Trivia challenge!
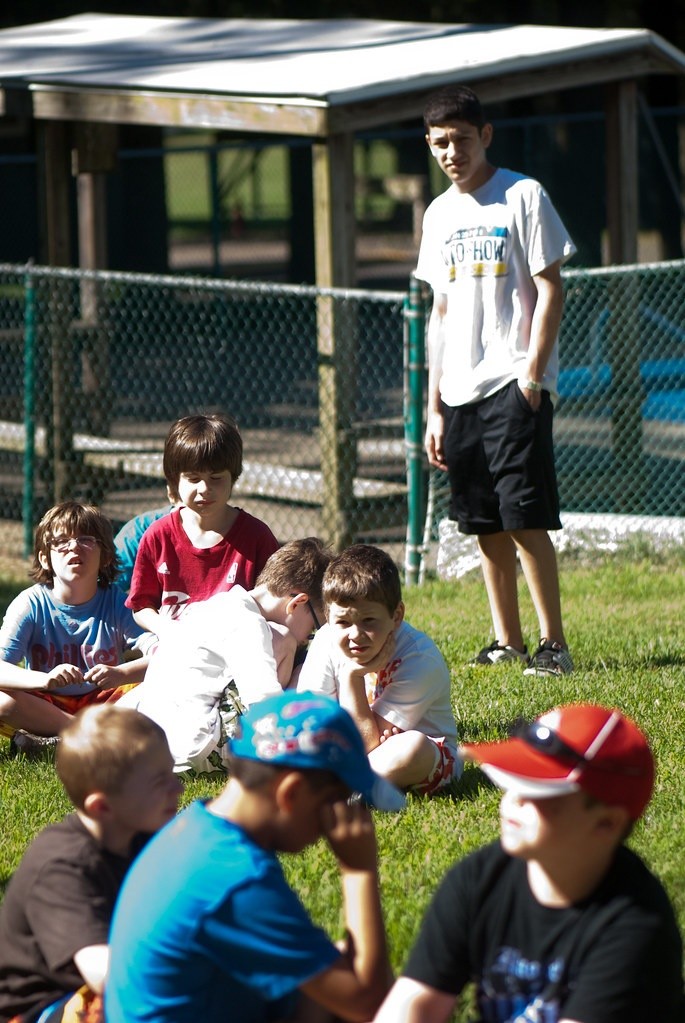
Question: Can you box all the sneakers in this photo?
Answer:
[523,637,573,678]
[462,640,530,669]
[13,728,62,764]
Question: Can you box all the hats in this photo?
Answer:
[461,703,655,822]
[229,689,407,813]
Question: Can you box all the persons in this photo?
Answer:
[102,691,395,1023]
[0,702,185,1023]
[0,502,158,765]
[373,703,685,1023]
[415,88,578,675]
[296,545,463,797]
[114,482,183,593]
[137,538,336,782]
[123,414,282,639]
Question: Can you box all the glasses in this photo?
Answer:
[47,536,107,552]
[506,717,643,777]
[290,593,321,640]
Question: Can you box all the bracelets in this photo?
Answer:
[517,379,541,392]
